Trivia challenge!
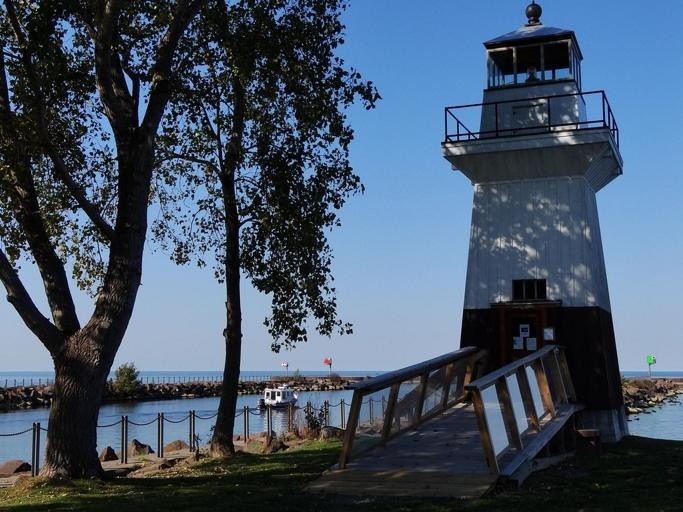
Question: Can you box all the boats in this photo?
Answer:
[258,384,298,410]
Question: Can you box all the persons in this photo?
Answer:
[525,66,540,83]
